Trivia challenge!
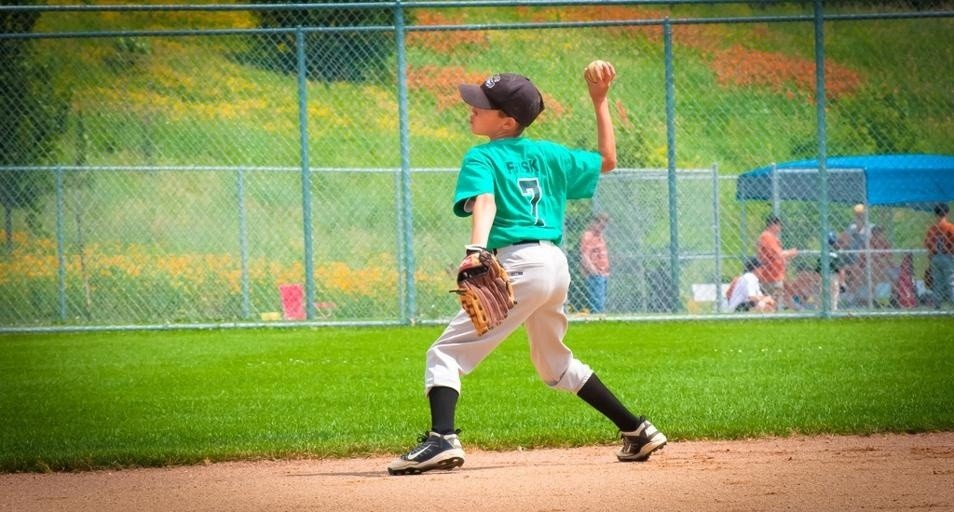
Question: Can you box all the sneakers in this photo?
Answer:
[616,415,667,462]
[388,429,465,475]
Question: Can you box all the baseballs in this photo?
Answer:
[588,61,610,83]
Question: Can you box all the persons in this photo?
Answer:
[925,204,954,311]
[580,213,610,314]
[728,212,900,313]
[388,62,667,476]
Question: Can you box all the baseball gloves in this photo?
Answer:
[457,244,515,335]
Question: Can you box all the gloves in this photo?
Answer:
[840,282,848,293]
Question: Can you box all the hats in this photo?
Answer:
[596,213,608,226]
[744,256,763,271]
[459,73,545,127]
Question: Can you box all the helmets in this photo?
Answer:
[854,204,866,214]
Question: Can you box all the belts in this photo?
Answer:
[513,240,539,245]
